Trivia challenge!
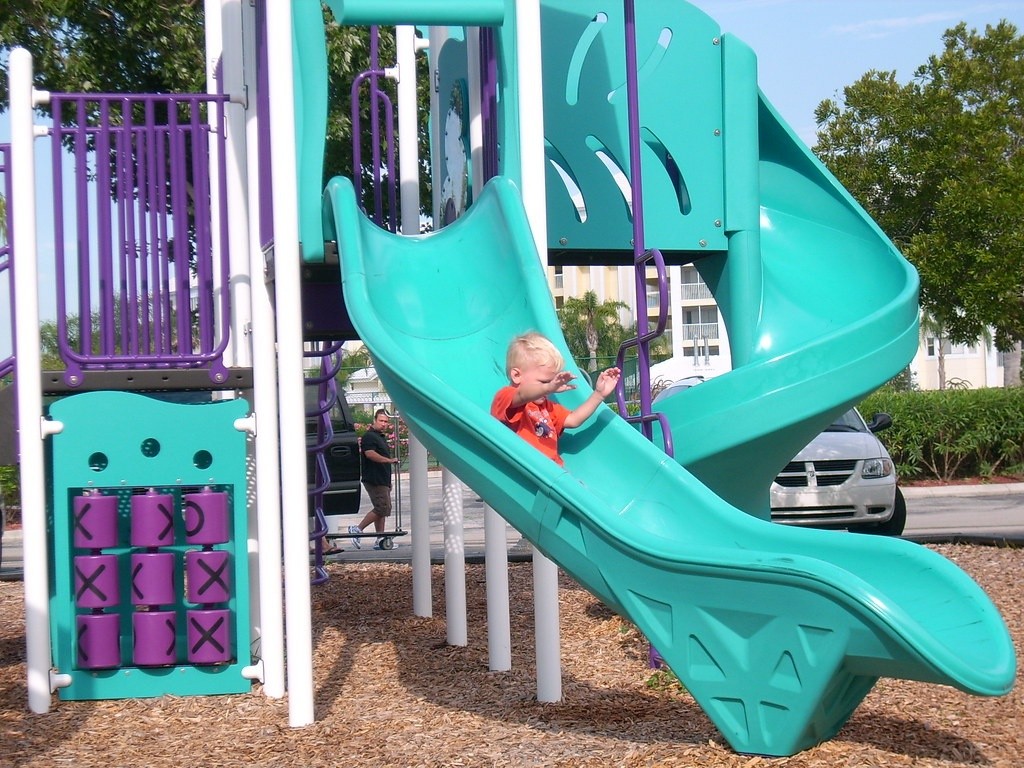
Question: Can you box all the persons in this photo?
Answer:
[347,409,400,550]
[309,516,344,555]
[490,333,621,468]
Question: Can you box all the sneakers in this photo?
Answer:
[373,538,400,549]
[348,524,364,549]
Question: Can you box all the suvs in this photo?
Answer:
[645,374,907,539]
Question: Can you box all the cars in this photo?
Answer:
[143,381,361,516]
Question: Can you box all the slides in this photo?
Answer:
[329,170,1020,759]
[630,82,927,523]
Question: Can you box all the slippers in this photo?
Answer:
[323,547,345,555]
